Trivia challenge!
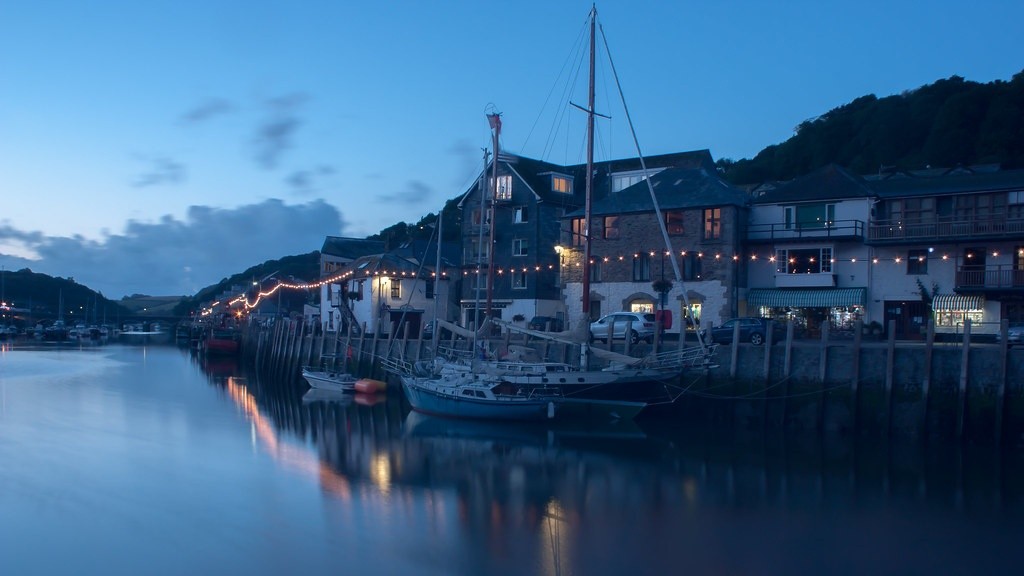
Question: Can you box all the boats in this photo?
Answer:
[303,372,360,393]
[377,333,726,421]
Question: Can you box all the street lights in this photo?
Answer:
[552,224,590,255]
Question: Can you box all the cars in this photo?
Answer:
[996,322,1024,341]
[424,320,465,339]
[589,311,663,344]
[697,316,785,346]
